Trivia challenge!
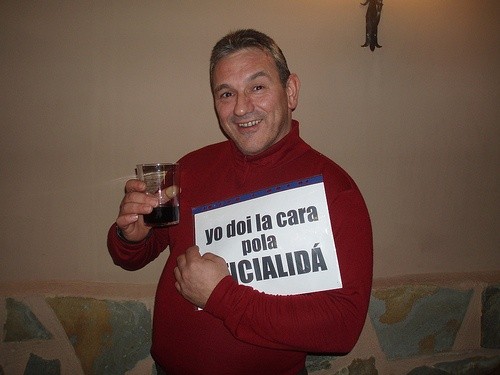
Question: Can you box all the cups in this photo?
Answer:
[135,163,180,227]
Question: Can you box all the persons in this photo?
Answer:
[107,27,373,375]
